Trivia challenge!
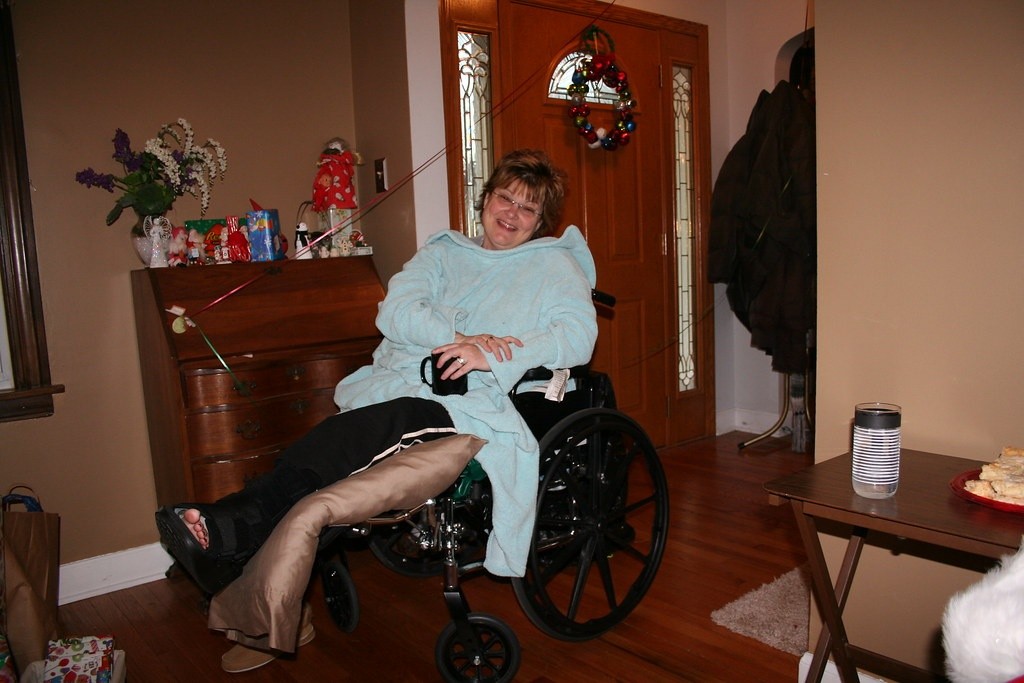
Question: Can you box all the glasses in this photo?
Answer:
[492,191,542,217]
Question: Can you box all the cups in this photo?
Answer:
[847,404,903,500]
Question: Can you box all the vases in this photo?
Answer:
[130,213,175,269]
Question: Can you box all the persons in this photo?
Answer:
[152,151,601,601]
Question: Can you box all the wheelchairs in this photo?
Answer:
[314,290,670,683]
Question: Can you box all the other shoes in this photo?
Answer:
[155,461,316,595]
[221,604,316,673]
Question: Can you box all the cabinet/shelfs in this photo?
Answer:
[129,253,388,510]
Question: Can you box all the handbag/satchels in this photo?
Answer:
[2,484,61,641]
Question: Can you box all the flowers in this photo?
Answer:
[75,118,227,226]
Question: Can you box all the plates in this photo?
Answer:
[948,464,1024,512]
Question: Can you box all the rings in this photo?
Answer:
[486,337,493,343]
[456,358,465,365]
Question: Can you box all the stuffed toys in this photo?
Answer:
[311,139,357,212]
[168,213,248,266]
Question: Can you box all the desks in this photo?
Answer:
[760,447,1024,683]
